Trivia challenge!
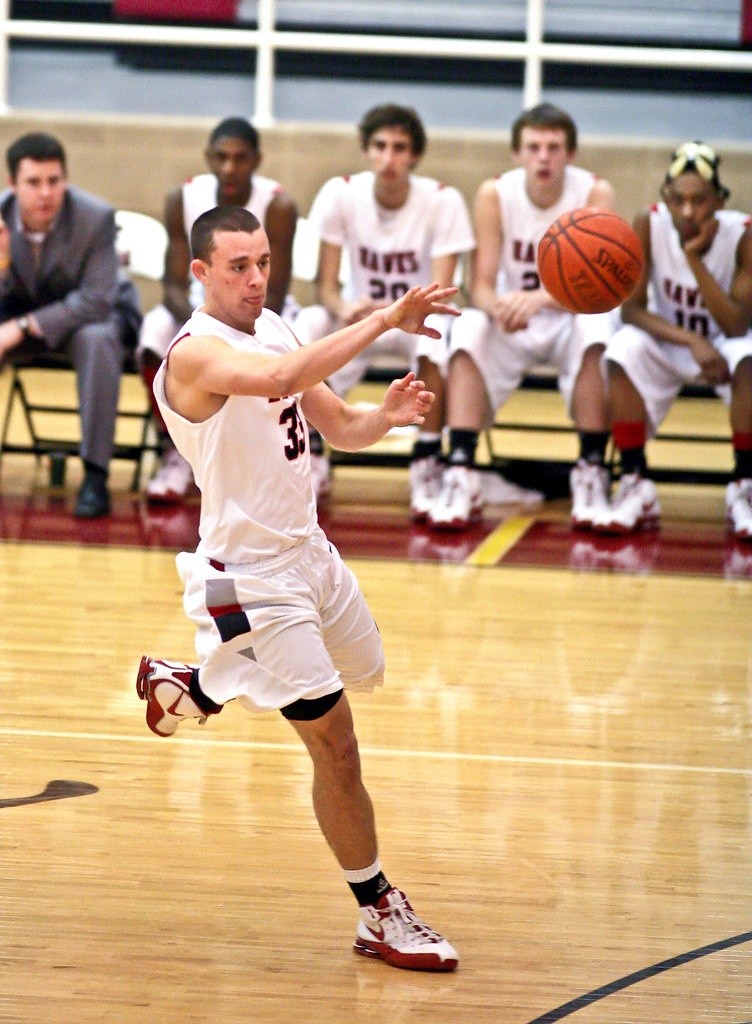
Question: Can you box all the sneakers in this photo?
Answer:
[311,454,331,499]
[570,462,611,525]
[411,459,444,514]
[591,475,660,531]
[145,450,194,500]
[724,478,752,537]
[135,655,224,738]
[429,465,481,527]
[353,887,459,970]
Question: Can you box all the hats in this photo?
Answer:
[665,142,720,188]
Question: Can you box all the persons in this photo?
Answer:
[297,104,616,532]
[136,205,462,973]
[0,116,297,520]
[608,141,752,542]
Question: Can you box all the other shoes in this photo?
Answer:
[71,475,108,516]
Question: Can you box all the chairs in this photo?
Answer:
[0,209,738,501]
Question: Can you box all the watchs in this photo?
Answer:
[16,314,30,338]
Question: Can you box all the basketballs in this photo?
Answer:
[537,205,645,315]
